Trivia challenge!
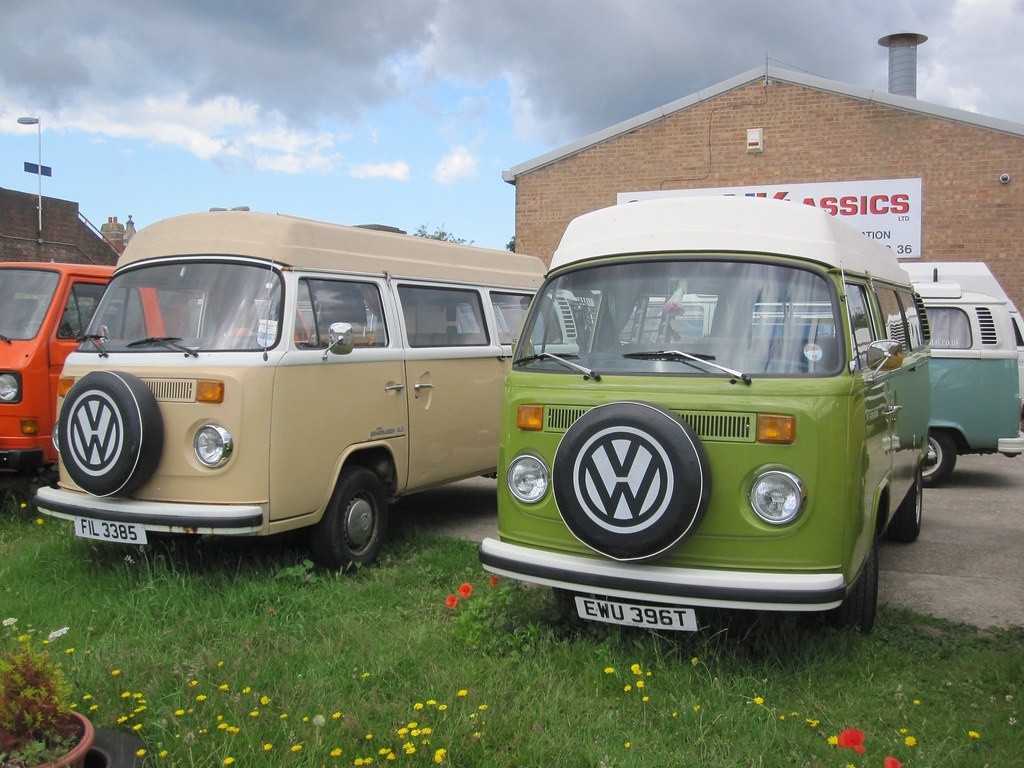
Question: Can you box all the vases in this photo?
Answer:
[0,709,95,768]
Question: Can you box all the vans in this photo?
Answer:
[35,211,560,573]
[1,260,309,482]
[471,195,935,635]
[616,281,1021,489]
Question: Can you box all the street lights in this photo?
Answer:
[16,117,44,244]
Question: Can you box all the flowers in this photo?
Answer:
[0,617,70,752]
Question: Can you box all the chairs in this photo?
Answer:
[400,303,416,347]
[416,304,449,348]
